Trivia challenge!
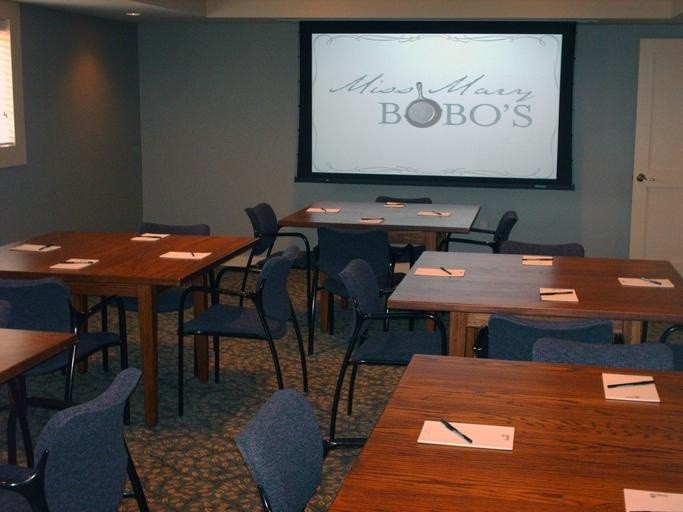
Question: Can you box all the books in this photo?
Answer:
[623,487,683,512]
[305,201,451,224]
[601,372,661,402]
[411,253,676,303]
[8,232,213,271]
[417,420,515,450]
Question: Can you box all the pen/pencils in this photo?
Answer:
[39,244,53,250]
[385,204,405,205]
[361,218,385,220]
[540,292,573,295]
[190,249,194,256]
[641,277,661,285]
[440,419,472,443]
[440,267,451,275]
[522,259,553,260]
[136,234,162,238]
[321,208,327,212]
[61,261,92,264]
[432,210,442,216]
[607,381,655,388]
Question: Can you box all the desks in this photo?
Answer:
[326,353,683,512]
[276,199,481,335]
[386,248,683,358]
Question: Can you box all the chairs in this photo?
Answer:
[533,335,677,370]
[438,209,519,315]
[486,312,614,361]
[501,239,585,257]
